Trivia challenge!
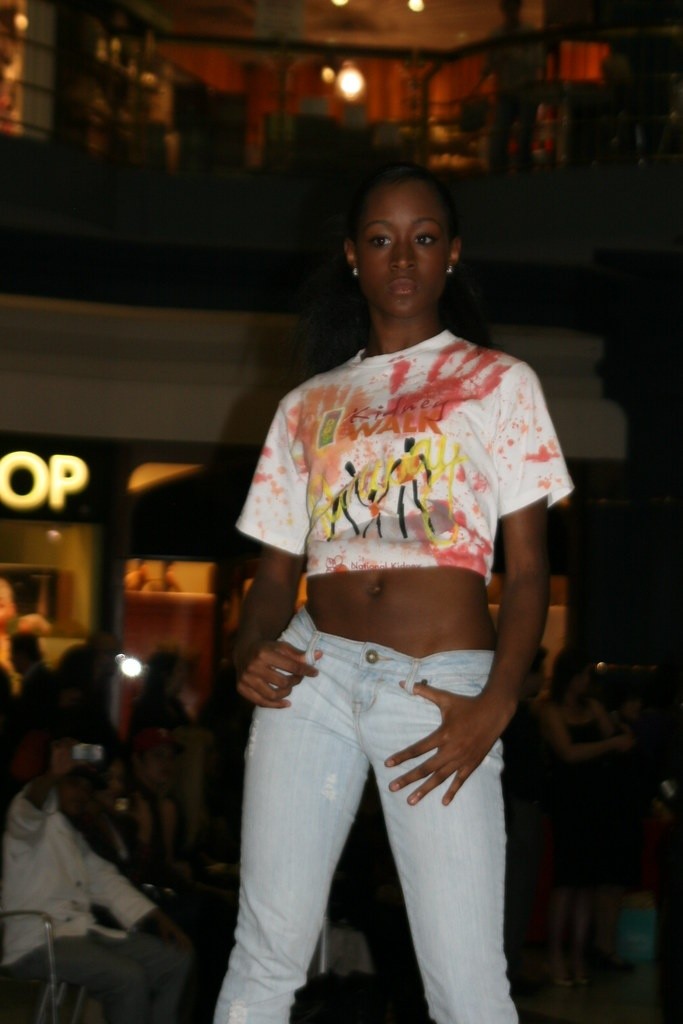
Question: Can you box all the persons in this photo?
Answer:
[211,172,575,1024]
[0,633,402,1024]
[486,0,544,172]
[506,647,683,984]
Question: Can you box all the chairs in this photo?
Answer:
[0,909,88,1024]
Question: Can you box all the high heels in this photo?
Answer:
[542,957,572,987]
[574,973,588,985]
